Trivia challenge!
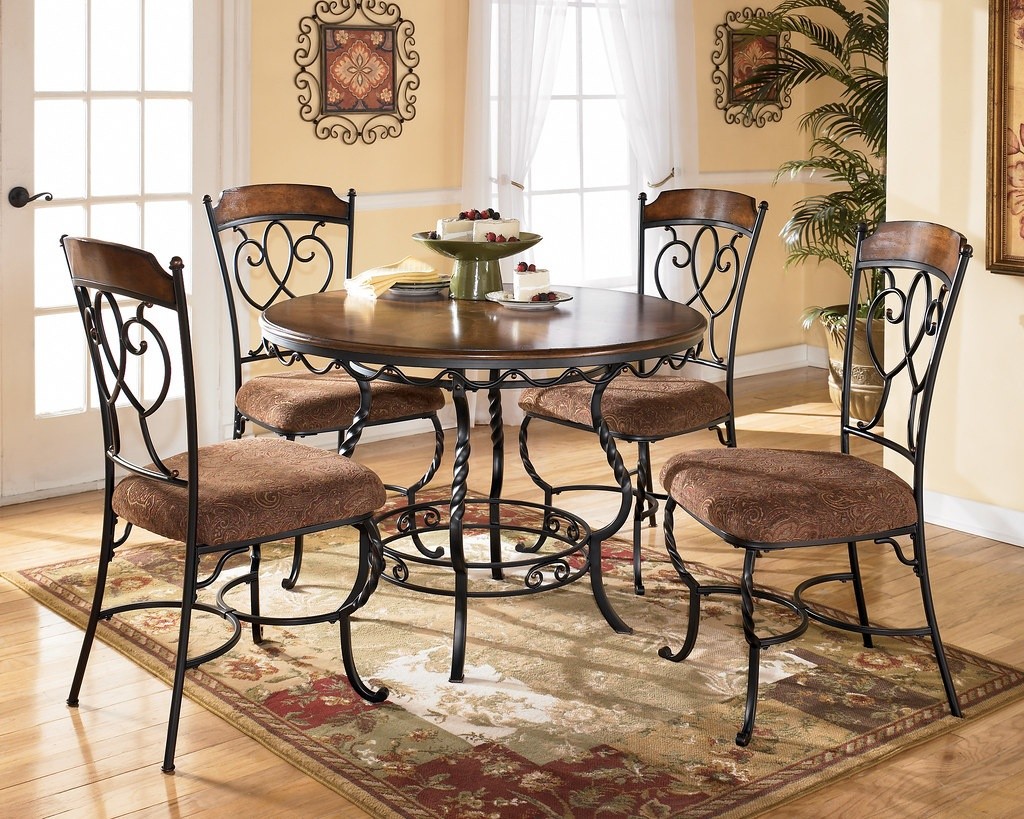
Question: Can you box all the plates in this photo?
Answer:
[388,273,450,296]
[485,290,573,310]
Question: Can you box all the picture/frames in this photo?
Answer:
[986,0,1024,277]
[710,6,794,129]
[294,1,420,146]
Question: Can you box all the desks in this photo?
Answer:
[257,277,707,682]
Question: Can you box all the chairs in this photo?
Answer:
[57,234,388,774]
[514,190,771,638]
[203,182,446,592]
[658,216,973,751]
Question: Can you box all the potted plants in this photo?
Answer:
[728,0,886,424]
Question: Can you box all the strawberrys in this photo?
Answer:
[485,232,520,242]
[532,292,556,301]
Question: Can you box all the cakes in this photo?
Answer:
[436,208,520,242]
[513,262,550,301]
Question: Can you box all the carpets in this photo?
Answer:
[1,480,1024,819]
